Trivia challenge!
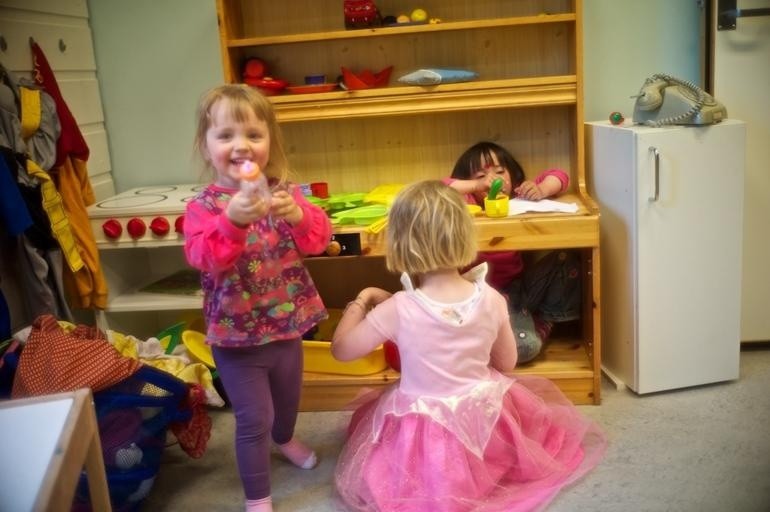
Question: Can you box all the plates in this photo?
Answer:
[290,84,338,95]
[181,329,216,369]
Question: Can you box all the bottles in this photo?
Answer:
[239,159,272,212]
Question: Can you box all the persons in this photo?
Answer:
[438,141,583,347]
[179,84,336,512]
[329,181,609,512]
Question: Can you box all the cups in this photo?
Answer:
[344,0,377,28]
[484,194,511,217]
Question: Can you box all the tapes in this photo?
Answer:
[305,75,325,85]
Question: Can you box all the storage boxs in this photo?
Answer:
[302,307,387,375]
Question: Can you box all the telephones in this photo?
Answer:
[633,74,728,125]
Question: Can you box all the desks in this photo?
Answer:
[0,387,113,512]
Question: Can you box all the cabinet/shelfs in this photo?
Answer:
[86,184,205,333]
[214,1,601,417]
[584,116,745,395]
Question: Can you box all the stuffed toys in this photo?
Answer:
[501,307,542,365]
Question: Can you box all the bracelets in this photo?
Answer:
[355,296,370,306]
